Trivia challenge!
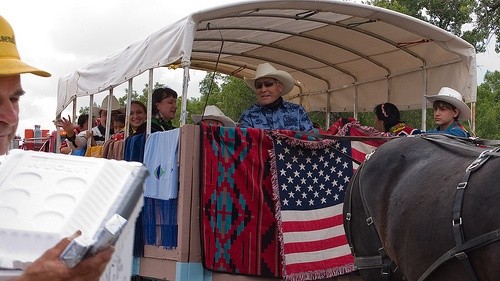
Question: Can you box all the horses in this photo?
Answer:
[342,130,500,281]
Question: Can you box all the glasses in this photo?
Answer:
[254,80,276,89]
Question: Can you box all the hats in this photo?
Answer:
[424,87,471,120]
[0,14,52,78]
[243,63,294,95]
[92,94,125,117]
[191,105,236,127]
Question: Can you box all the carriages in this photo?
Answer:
[54,0,500,280]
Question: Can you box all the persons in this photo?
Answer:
[111,101,147,140]
[113,114,125,134]
[55,94,121,150]
[193,105,236,127]
[423,87,472,138]
[133,88,177,136]
[0,16,114,281]
[374,103,421,136]
[235,63,314,132]
